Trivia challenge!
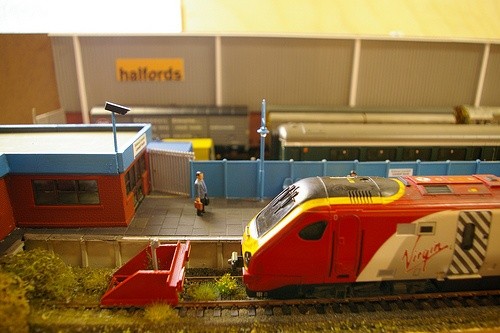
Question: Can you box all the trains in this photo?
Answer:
[242,174,500,296]
[279,123,500,162]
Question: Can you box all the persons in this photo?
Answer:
[194,171,207,217]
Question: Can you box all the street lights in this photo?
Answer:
[259,99,267,202]
